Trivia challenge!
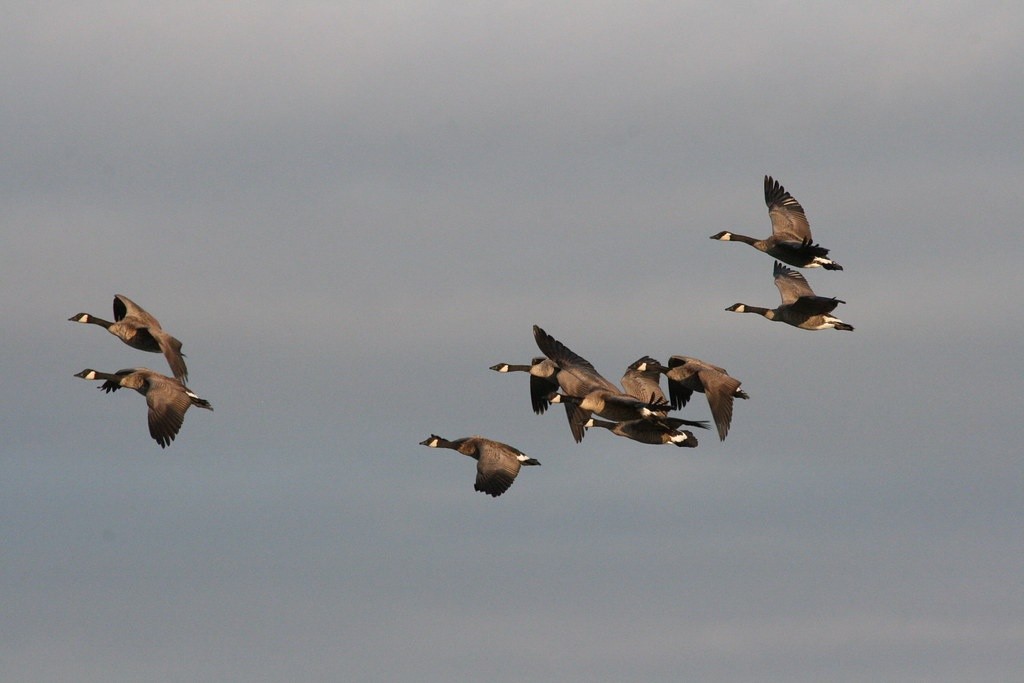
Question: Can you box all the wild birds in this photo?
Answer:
[418,431,544,497]
[68,291,213,449]
[709,172,855,332]
[488,322,750,448]
